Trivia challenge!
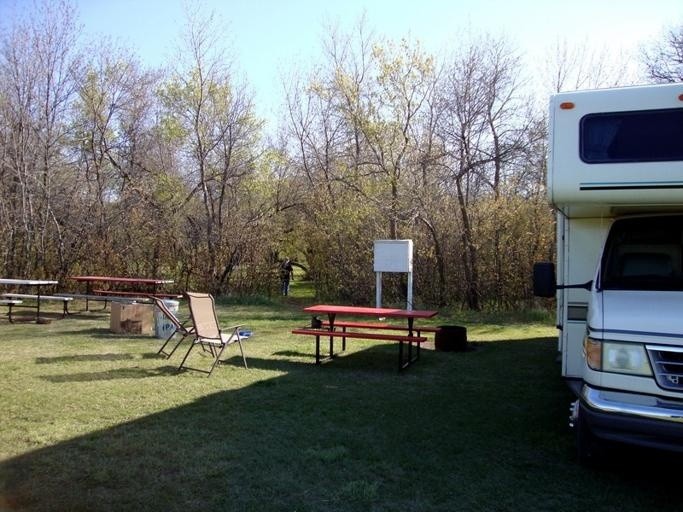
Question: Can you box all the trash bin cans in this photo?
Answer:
[153,300,179,339]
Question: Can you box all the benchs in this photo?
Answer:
[0,292,73,319]
[290,328,426,374]
[52,293,150,316]
[0,299,24,325]
[92,288,184,310]
[321,320,442,360]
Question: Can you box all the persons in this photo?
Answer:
[277,257,294,296]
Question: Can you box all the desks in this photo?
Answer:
[67,275,175,312]
[304,304,438,373]
[0,278,58,322]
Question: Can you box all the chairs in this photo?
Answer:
[176,290,248,378]
[148,294,214,360]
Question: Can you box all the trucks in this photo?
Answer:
[534,82,682,453]
